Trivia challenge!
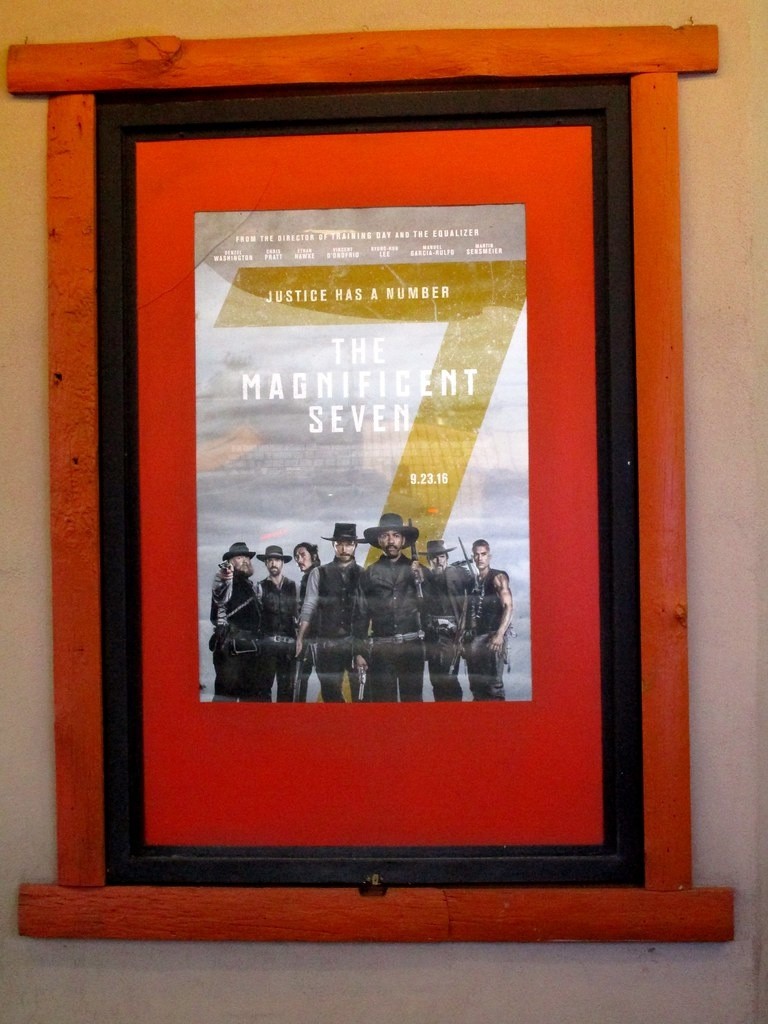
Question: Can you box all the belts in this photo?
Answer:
[372,631,420,644]
[262,635,296,644]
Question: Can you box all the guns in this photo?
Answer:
[357,666,368,701]
[218,560,230,572]
[288,517,510,702]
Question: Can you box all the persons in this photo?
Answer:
[352,514,449,702]
[251,545,298,702]
[460,539,514,701]
[291,542,322,699]
[209,543,263,701]
[419,540,474,701]
[295,522,372,703]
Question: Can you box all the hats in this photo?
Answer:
[418,539,457,556]
[320,523,368,543]
[364,513,420,549]
[256,545,293,563]
[222,542,256,562]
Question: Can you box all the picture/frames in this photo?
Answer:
[3,25,734,946]
